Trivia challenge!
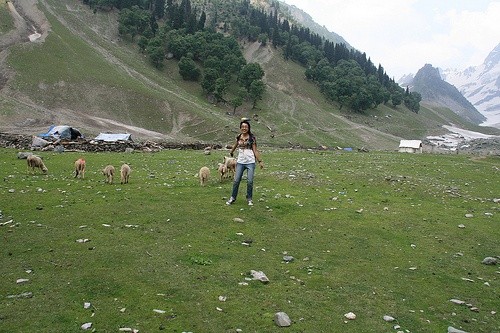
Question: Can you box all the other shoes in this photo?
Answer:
[226,197,235,204]
[248,200,253,206]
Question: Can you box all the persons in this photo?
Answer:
[226,121,264,206]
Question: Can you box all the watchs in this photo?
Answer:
[258,161,261,163]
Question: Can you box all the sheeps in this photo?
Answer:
[102,164,116,185]
[119,163,131,184]
[27,154,48,174]
[216,156,237,183]
[198,166,211,186]
[73,157,86,180]
